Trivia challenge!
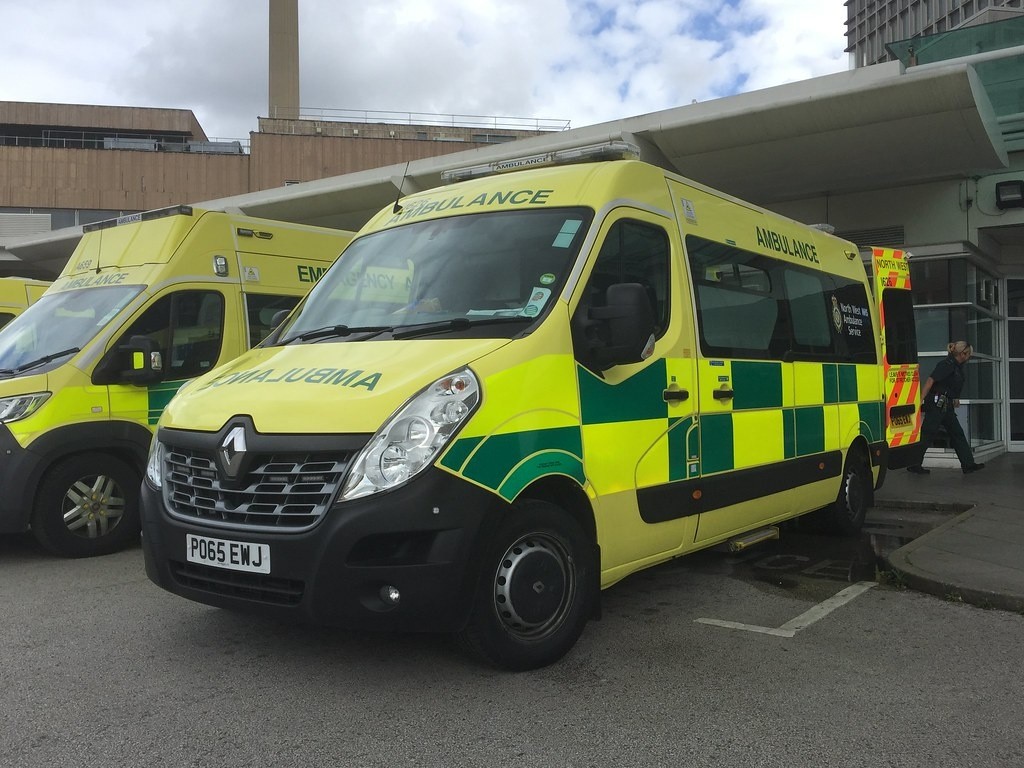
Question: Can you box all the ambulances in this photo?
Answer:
[1,276,97,371]
[1,202,415,560]
[137,141,923,672]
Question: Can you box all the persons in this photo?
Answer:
[906,340,985,475]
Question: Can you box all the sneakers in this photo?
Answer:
[907,465,930,474]
[962,463,984,474]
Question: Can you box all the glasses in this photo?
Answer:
[962,343,970,352]
